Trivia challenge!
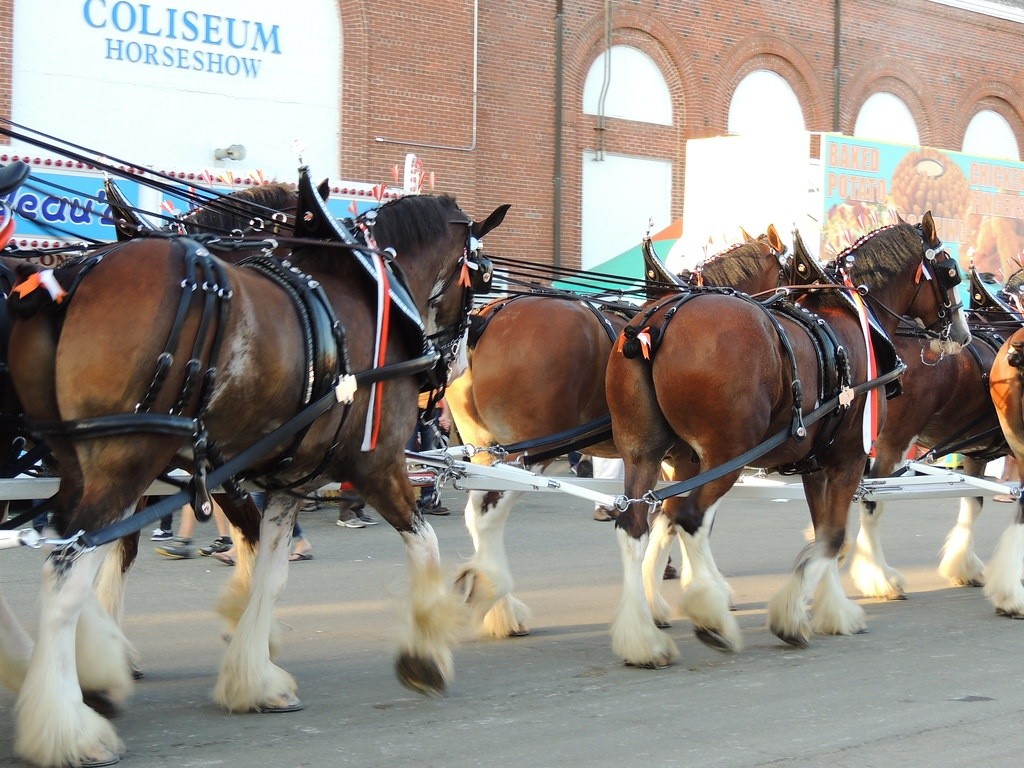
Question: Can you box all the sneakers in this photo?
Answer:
[422,504,450,514]
[150,527,174,540]
[156,540,196,559]
[358,515,379,525]
[196,537,233,557]
[336,518,367,528]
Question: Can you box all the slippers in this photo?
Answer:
[288,552,314,561]
[213,553,236,566]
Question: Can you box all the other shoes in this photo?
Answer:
[19,528,44,547]
[571,463,579,475]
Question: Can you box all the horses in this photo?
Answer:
[0,178,1024,768]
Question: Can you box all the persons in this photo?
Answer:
[568,450,621,521]
[32,461,58,533]
[150,491,379,567]
[418,387,451,520]
[0,159,30,198]
